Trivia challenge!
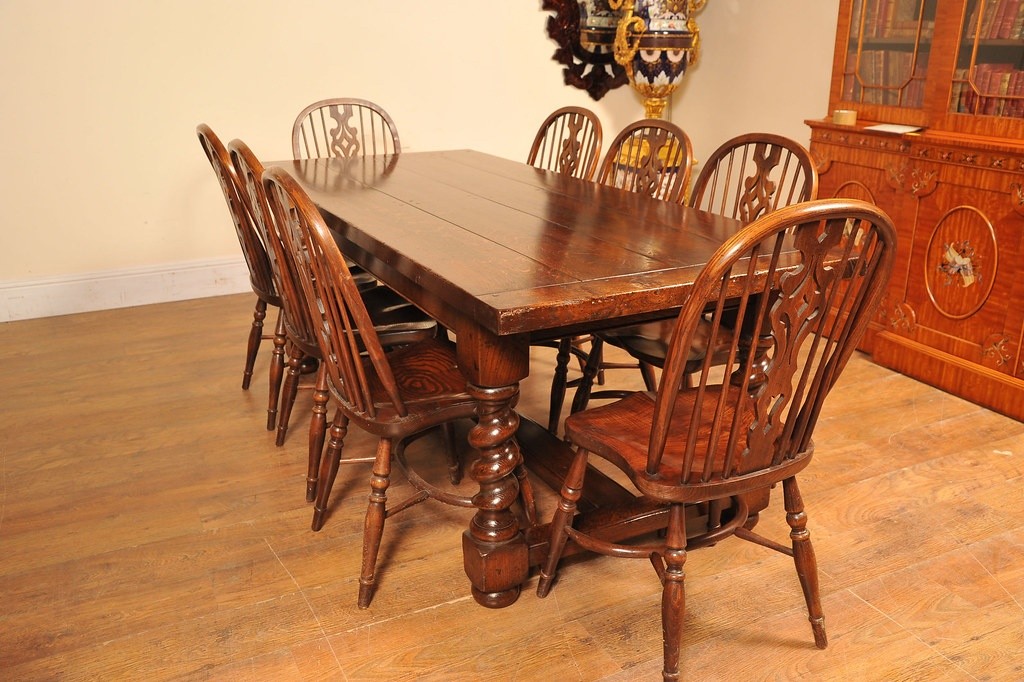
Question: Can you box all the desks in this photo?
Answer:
[269,145,864,610]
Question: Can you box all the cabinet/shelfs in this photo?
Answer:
[782,0,1024,425]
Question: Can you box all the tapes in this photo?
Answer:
[832,109,858,126]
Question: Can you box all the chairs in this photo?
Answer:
[194,98,897,681]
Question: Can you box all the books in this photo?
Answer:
[948,0,1024,117]
[841,0,935,108]
